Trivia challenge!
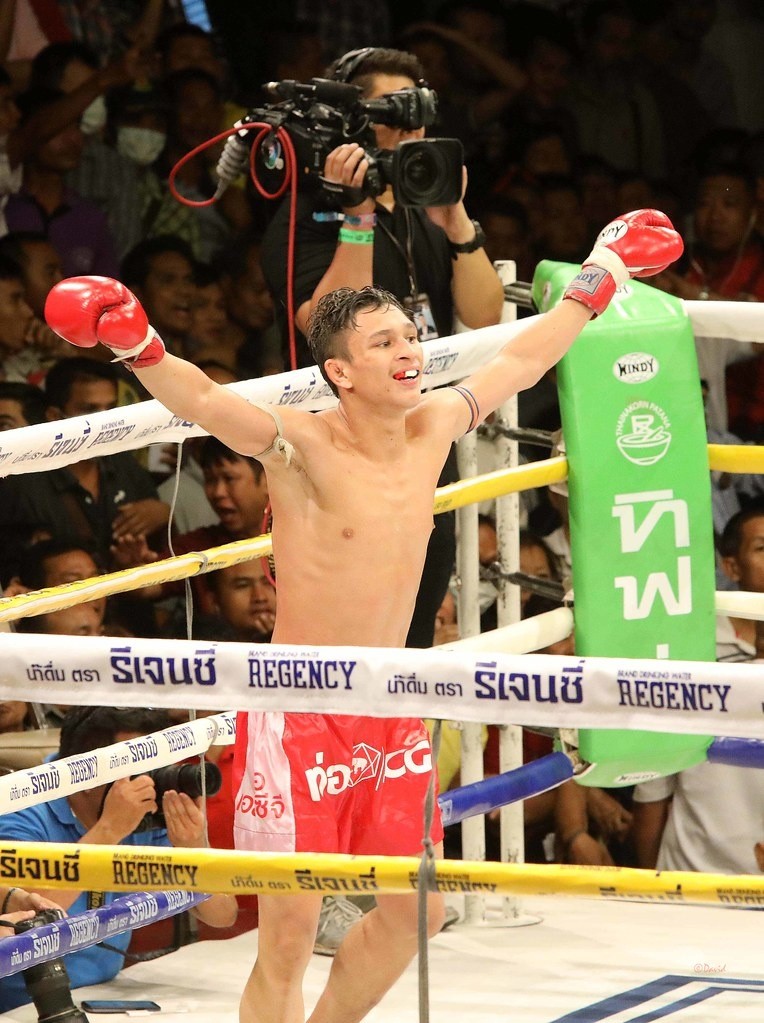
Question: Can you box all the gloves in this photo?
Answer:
[564,207,684,320]
[45,274,166,370]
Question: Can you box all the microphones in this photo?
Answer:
[266,76,364,106]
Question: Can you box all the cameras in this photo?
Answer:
[12,907,90,1023]
[126,758,222,833]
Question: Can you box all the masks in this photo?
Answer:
[118,127,167,163]
[79,96,108,133]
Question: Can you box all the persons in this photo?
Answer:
[257,48,506,960]
[0,0,764,964]
[0,706,238,1014]
[45,207,684,1021]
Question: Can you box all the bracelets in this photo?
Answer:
[342,214,377,226]
[3,888,15,914]
[696,285,710,300]
[337,228,374,244]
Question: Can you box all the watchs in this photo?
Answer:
[447,220,487,261]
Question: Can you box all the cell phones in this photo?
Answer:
[81,999,162,1013]
[177,0,214,34]
